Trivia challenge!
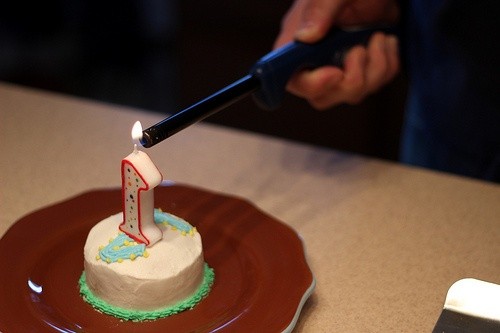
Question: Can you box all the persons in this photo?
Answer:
[274,1,499,184]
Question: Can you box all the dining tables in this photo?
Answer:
[0,85,500,332]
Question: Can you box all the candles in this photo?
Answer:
[118,119,163,247]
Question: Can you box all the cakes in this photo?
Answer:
[79,205,215,323]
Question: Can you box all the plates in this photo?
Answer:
[0,180,314,333]
